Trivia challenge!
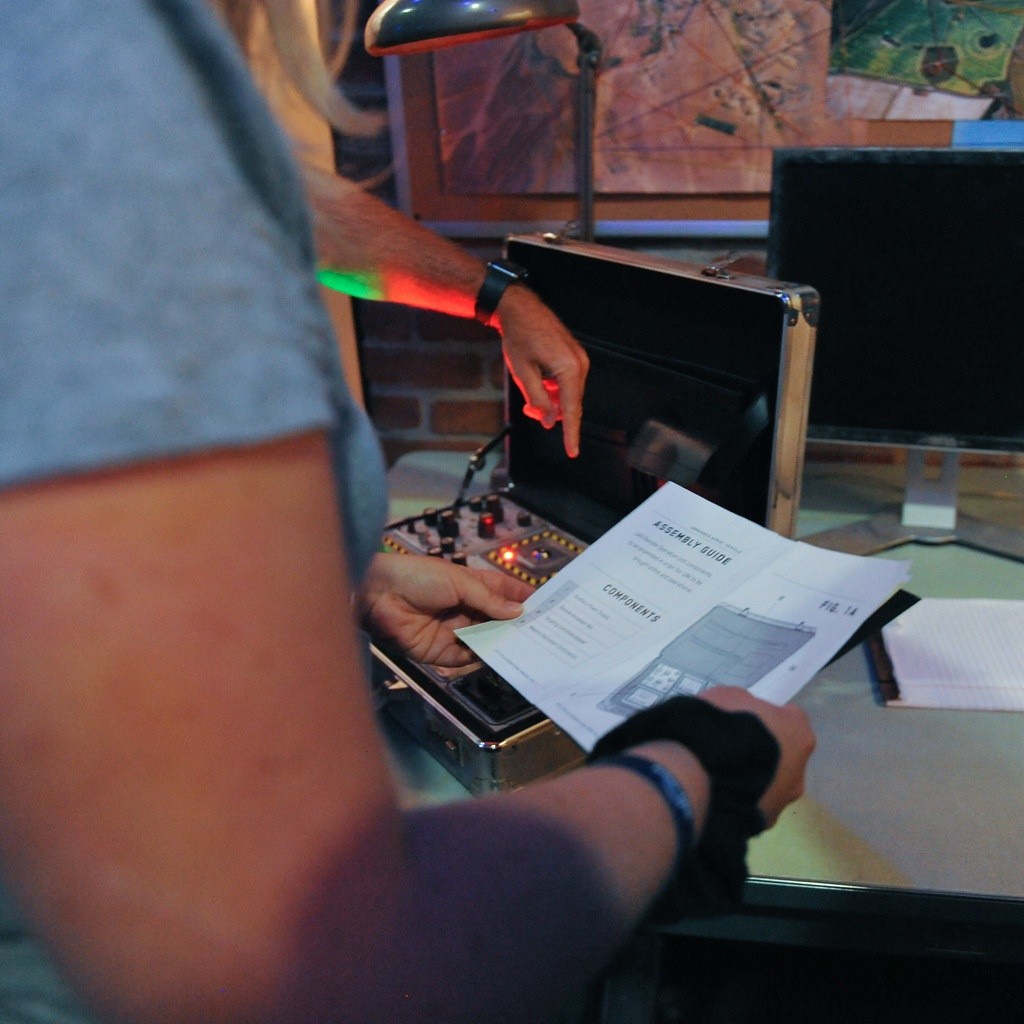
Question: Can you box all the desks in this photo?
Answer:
[382,450,1024,927]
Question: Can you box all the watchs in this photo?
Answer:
[475,256,529,325]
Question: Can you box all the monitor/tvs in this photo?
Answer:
[767,140,1024,568]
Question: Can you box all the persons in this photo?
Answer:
[0,0,815,1024]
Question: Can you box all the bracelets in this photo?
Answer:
[591,691,783,890]
[581,745,702,880]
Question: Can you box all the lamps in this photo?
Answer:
[363,0,603,241]
